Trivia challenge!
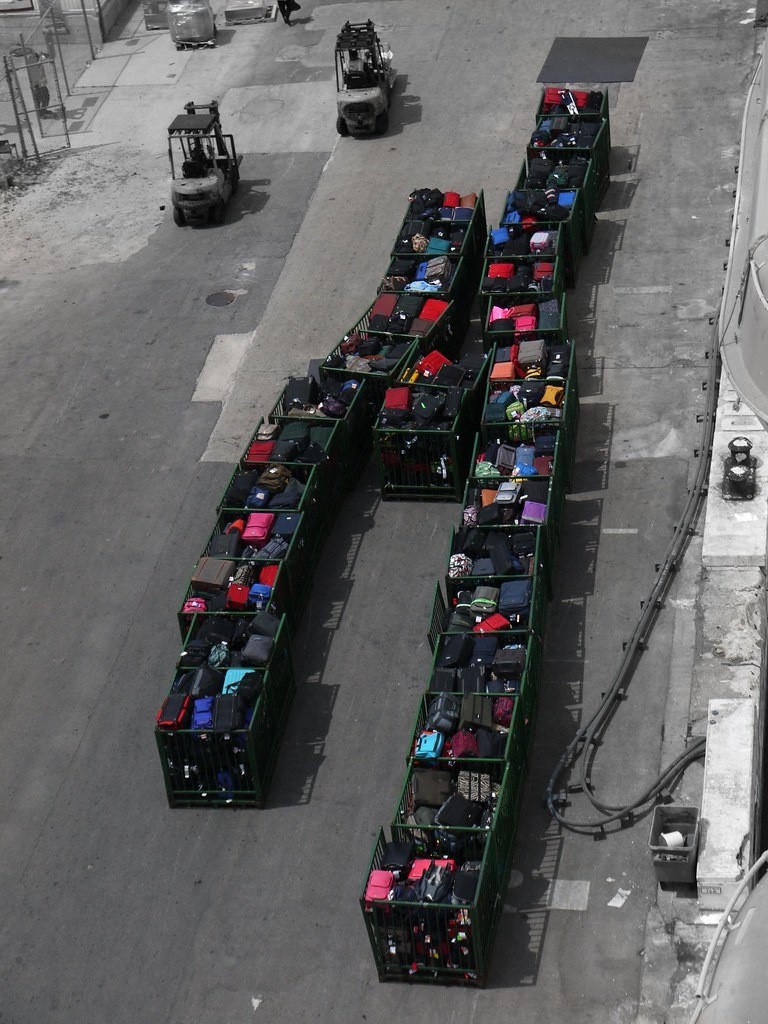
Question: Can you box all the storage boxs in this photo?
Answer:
[152,84,614,991]
[648,804,700,890]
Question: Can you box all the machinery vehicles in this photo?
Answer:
[334,18,398,136]
[166,100,243,228]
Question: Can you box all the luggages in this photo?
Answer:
[152,84,612,992]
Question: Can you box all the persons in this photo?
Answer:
[26,52,53,120]
[275,0,302,26]
[345,50,369,78]
